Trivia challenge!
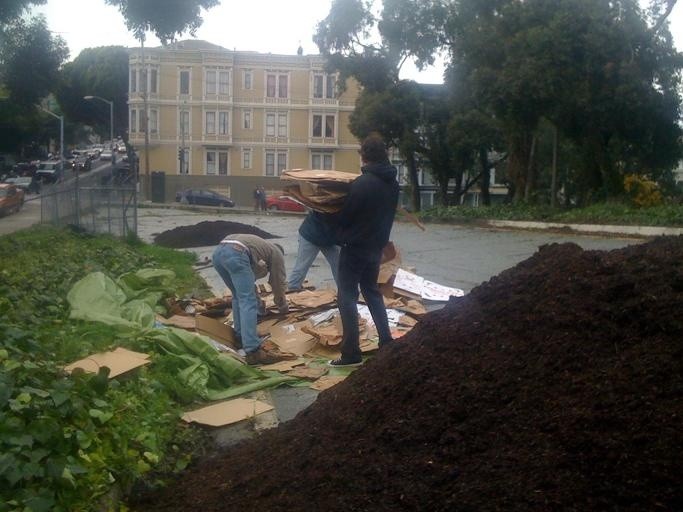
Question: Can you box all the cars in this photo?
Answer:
[0,182,25,216]
[175,185,236,207]
[4,134,127,192]
[265,193,306,213]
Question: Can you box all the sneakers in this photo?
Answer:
[245,349,282,366]
[325,355,362,367]
[234,336,242,349]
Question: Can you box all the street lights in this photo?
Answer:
[84,95,114,163]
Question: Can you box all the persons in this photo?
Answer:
[288,210,341,292]
[254,185,267,211]
[327,134,400,367]
[212,232,288,365]
